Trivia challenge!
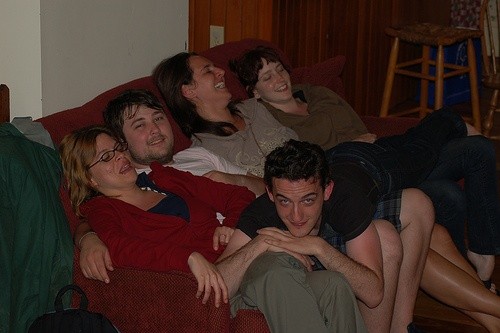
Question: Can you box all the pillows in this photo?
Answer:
[292,55,346,86]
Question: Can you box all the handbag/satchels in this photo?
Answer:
[28,284,120,333]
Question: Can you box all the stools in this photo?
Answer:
[379,23,483,133]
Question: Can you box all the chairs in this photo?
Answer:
[480,0,500,141]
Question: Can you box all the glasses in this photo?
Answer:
[86,140,128,171]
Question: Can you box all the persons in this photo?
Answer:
[58,45,500,333]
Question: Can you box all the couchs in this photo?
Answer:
[0,38,476,333]
